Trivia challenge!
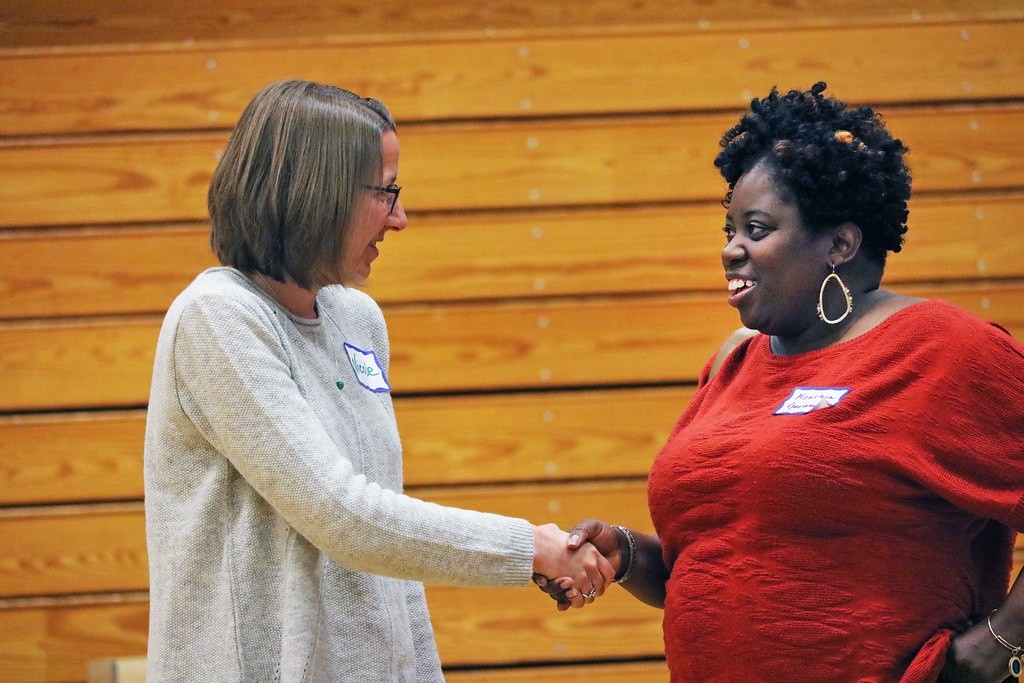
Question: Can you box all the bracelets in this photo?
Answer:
[608,525,638,585]
[987,609,1024,678]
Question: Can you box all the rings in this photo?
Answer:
[582,587,596,599]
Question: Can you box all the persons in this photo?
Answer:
[534,88,1024,683]
[143,79,615,683]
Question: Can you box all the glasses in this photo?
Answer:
[363,184,402,214]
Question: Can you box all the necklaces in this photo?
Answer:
[257,271,290,310]
